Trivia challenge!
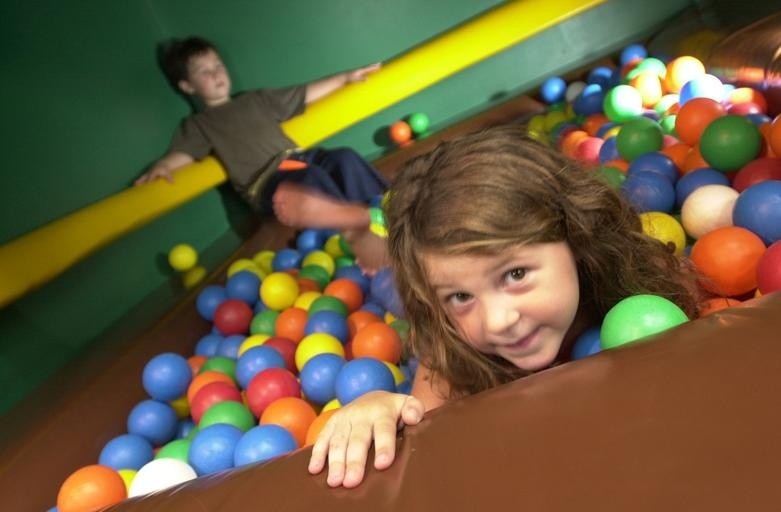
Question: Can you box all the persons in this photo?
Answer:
[132,35,382,232]
[308,126,714,489]
[525,43,781,361]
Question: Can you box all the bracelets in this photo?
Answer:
[346,71,352,85]
